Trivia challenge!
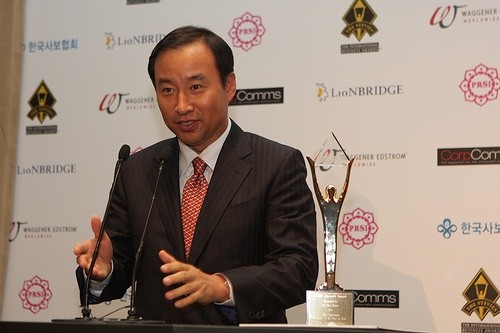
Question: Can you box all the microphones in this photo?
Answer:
[83,144,130,319]
[128,149,171,321]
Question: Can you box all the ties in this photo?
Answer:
[181,158,210,262]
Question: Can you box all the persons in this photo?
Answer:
[74,25,319,327]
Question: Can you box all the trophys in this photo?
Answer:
[306,130,356,326]
